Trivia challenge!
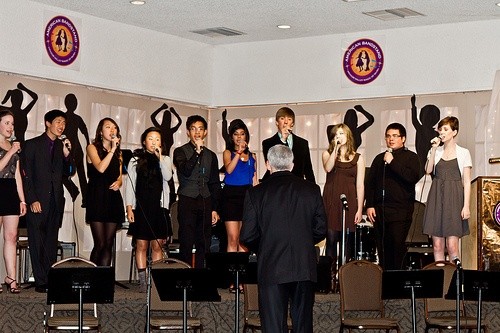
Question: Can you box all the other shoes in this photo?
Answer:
[138,271,147,293]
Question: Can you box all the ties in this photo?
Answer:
[49,142,54,160]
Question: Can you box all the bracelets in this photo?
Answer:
[110,150,115,153]
[237,151,242,154]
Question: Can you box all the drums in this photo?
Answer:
[349,214,377,261]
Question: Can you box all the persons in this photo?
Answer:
[86,117,126,267]
[365,122,425,270]
[262,106,316,182]
[0,105,27,293]
[172,114,220,267]
[220,124,258,294]
[238,144,327,333]
[124,127,173,291]
[322,123,365,293]
[20,109,75,293]
[424,115,472,265]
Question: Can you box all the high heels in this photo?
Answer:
[5,276,21,294]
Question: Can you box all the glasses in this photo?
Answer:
[386,134,403,139]
[188,127,205,131]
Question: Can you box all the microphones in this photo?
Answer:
[337,138,340,144]
[10,136,21,157]
[452,255,463,271]
[340,194,349,212]
[196,137,204,150]
[384,148,393,165]
[431,135,443,145]
[288,129,293,135]
[112,135,119,146]
[154,147,159,152]
[239,139,249,150]
[61,135,70,151]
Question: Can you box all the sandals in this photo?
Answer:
[229,287,245,294]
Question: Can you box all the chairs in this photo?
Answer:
[43,257,101,333]
[405,202,436,269]
[129,236,152,285]
[144,258,204,333]
[243,285,293,333]
[167,201,197,250]
[338,260,402,333]
[16,215,64,288]
[422,261,485,333]
